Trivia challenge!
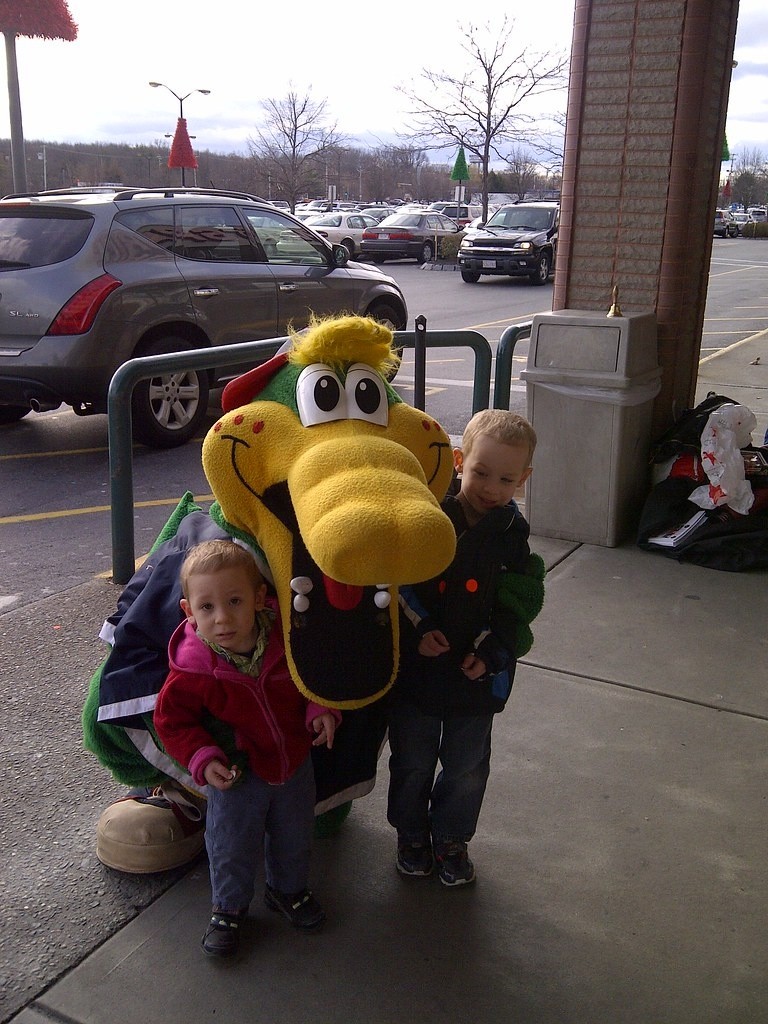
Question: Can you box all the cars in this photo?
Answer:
[217,212,294,255]
[731,206,767,232]
[359,210,457,263]
[276,213,379,261]
[293,199,516,223]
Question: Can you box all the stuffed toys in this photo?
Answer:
[82,320,453,876]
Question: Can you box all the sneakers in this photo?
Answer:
[432,832,475,887]
[265,881,327,933]
[396,828,433,876]
[200,905,248,958]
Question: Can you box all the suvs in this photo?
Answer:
[714,210,740,239]
[0,189,406,449]
[265,199,291,213]
[441,206,493,228]
[457,198,561,284]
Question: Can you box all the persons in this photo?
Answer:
[154,539,343,956]
[383,408,545,886]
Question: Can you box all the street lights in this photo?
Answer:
[164,134,197,185]
[325,137,361,198]
[149,81,210,186]
[449,125,477,225]
